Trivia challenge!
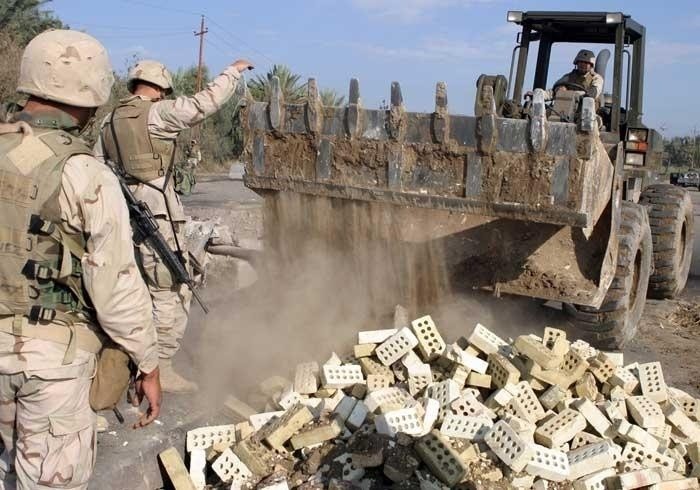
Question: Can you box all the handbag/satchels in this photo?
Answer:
[89,347,130,412]
[174,166,196,196]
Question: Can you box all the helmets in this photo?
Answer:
[127,59,174,95]
[15,29,115,107]
[573,49,595,68]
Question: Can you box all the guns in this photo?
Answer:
[108,160,208,316]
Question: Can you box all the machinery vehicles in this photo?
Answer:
[239,12,695,349]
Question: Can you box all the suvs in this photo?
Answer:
[670,168,700,192]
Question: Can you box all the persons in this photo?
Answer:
[92,59,255,395]
[185,140,201,174]
[554,50,603,113]
[0,28,161,490]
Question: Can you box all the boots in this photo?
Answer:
[157,358,198,395]
